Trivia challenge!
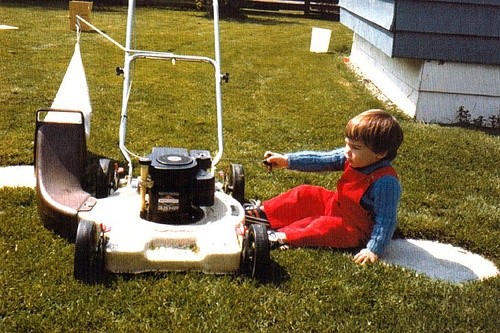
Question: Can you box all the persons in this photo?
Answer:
[243,108,405,267]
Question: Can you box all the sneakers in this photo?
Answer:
[243,198,267,226]
[250,229,280,250]
